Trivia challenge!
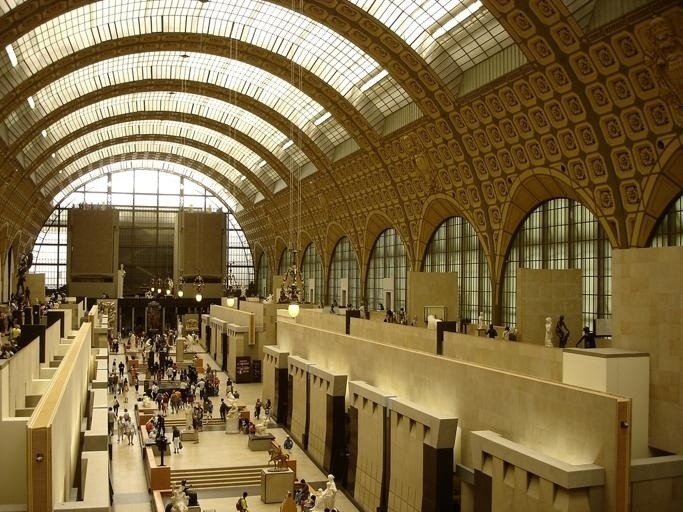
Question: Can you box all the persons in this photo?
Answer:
[501,326,510,340]
[107,323,272,454]
[294,478,316,511]
[331,509,336,512]
[574,326,597,348]
[544,316,553,343]
[378,302,406,323]
[323,507,329,511]
[181,478,199,506]
[478,311,486,328]
[322,473,337,502]
[283,434,293,454]
[358,302,364,318]
[238,491,249,511]
[1,312,16,337]
[486,324,498,338]
[375,506,383,511]
[555,314,570,348]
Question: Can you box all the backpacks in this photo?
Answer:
[236,500,241,510]
[265,409,269,414]
[287,442,291,449]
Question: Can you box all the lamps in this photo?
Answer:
[192,2,205,303]
[280,3,303,318]
[222,2,238,308]
[148,0,187,297]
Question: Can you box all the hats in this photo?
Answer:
[287,435,289,437]
[505,327,509,330]
[173,390,175,392]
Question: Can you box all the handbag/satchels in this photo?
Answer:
[179,442,182,449]
[164,403,167,407]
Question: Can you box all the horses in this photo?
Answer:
[267,449,289,469]
